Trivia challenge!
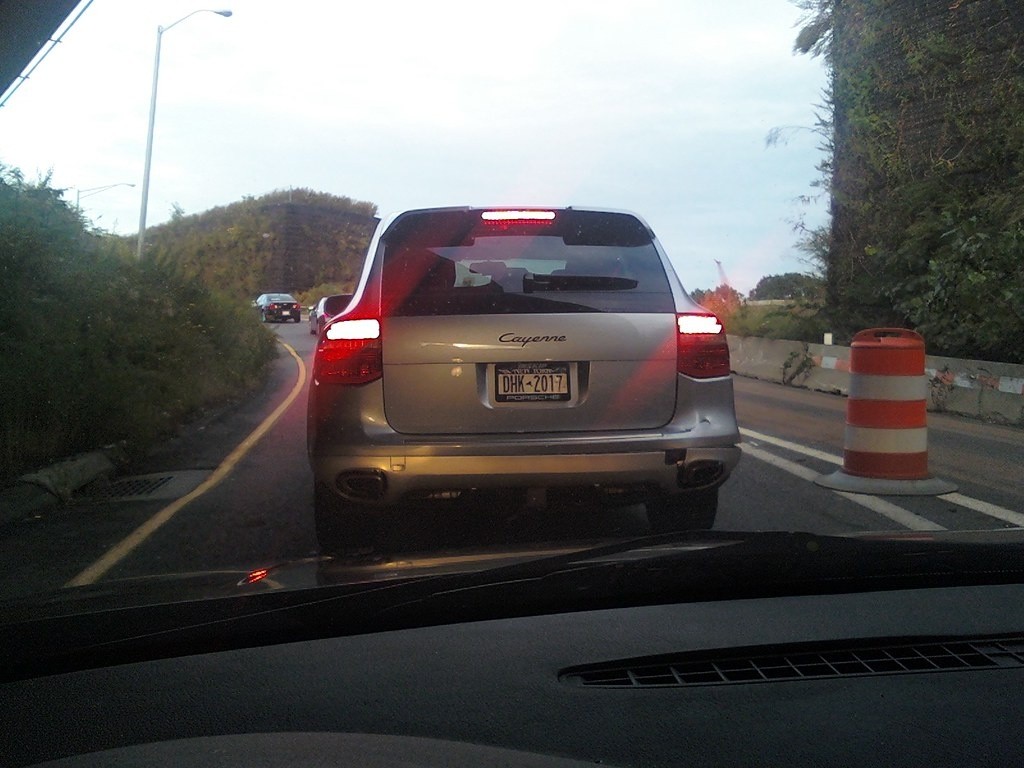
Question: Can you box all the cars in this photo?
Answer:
[300,207,745,542]
[253,293,300,324]
[307,291,354,336]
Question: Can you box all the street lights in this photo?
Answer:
[135,8,233,267]
[75,183,134,225]
